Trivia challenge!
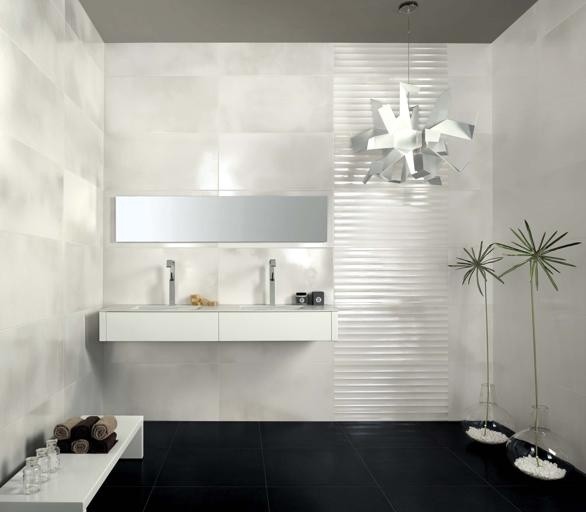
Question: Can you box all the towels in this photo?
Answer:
[49,415,118,454]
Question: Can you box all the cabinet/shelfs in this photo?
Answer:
[98,195,340,343]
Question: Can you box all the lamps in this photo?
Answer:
[363,0,475,186]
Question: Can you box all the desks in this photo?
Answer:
[0,414,145,512]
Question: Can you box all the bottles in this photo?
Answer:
[23,440,61,493]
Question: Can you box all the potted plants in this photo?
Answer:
[493,219,582,482]
[447,240,517,444]
